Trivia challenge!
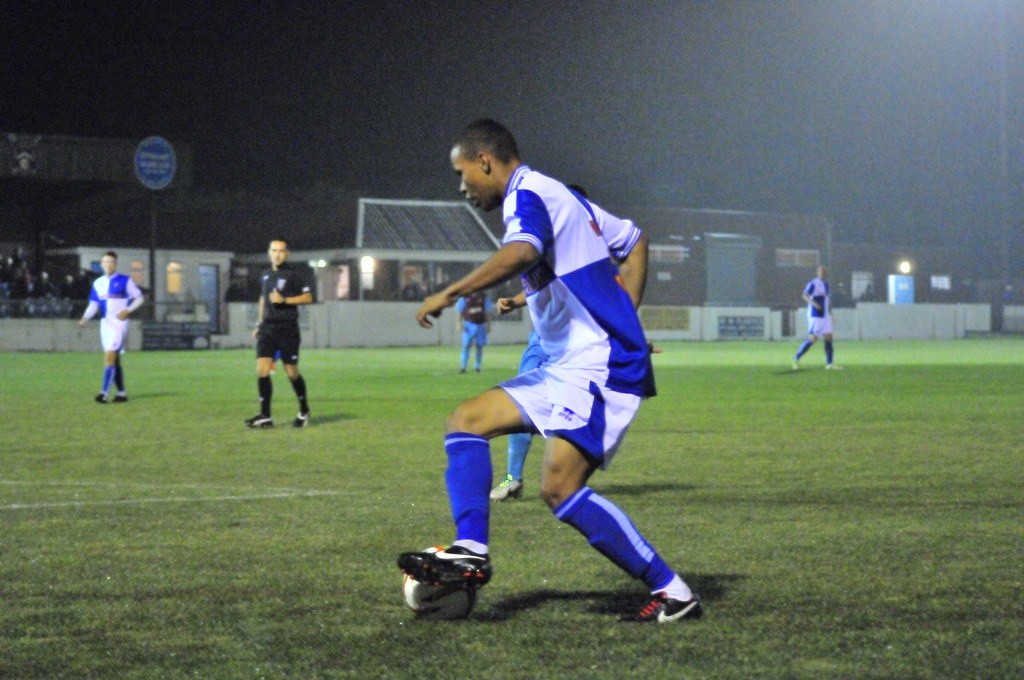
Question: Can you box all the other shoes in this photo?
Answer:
[825,364,843,370]
[95,392,109,404]
[792,357,799,370]
[112,394,127,403]
[475,369,481,373]
[458,368,467,374]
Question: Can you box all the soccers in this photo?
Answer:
[402,544,477,620]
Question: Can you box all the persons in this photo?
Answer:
[491,186,626,501]
[270,352,282,373]
[78,250,144,404]
[0,264,94,298]
[395,119,702,625]
[455,291,492,373]
[793,265,843,372]
[245,238,317,429]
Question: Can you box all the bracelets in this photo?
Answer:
[282,296,286,304]
[255,321,261,327]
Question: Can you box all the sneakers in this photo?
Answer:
[490,481,524,503]
[245,414,274,428]
[618,591,704,625]
[293,411,310,428]
[397,545,492,586]
